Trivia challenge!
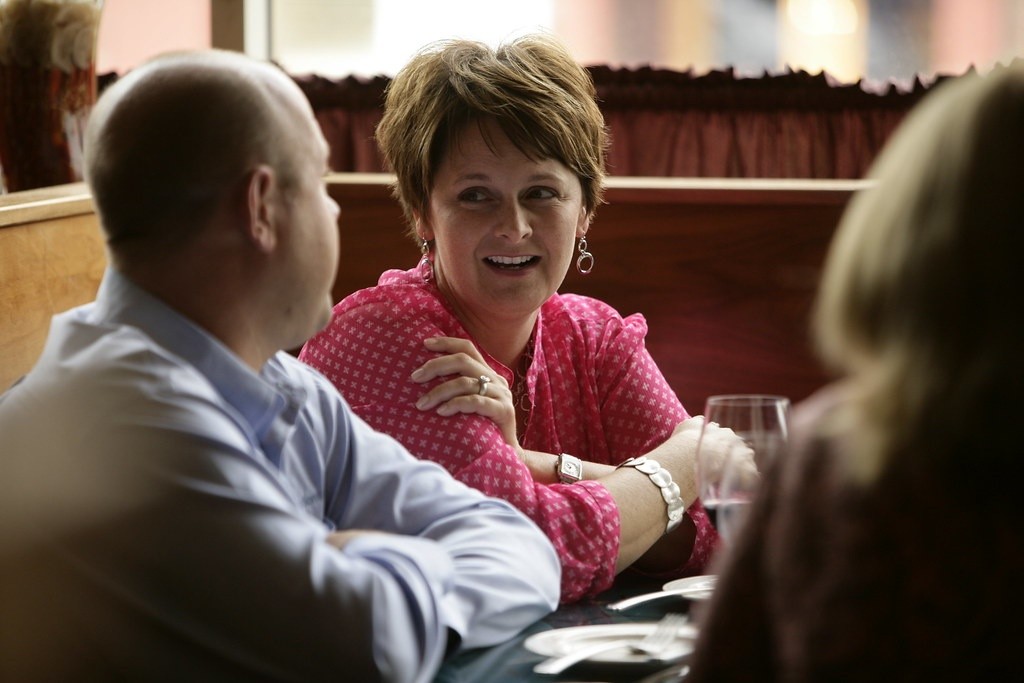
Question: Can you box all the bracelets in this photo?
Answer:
[615,456,683,535]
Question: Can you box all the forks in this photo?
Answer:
[532,613,688,674]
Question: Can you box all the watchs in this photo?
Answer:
[556,452,583,484]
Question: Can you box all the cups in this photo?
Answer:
[693,391,790,559]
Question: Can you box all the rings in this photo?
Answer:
[478,375,490,396]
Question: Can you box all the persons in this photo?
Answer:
[682,64,1023,683]
[297,34,759,602]
[0,48,561,683]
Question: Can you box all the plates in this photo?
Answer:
[662,574,719,600]
[524,623,700,664]
